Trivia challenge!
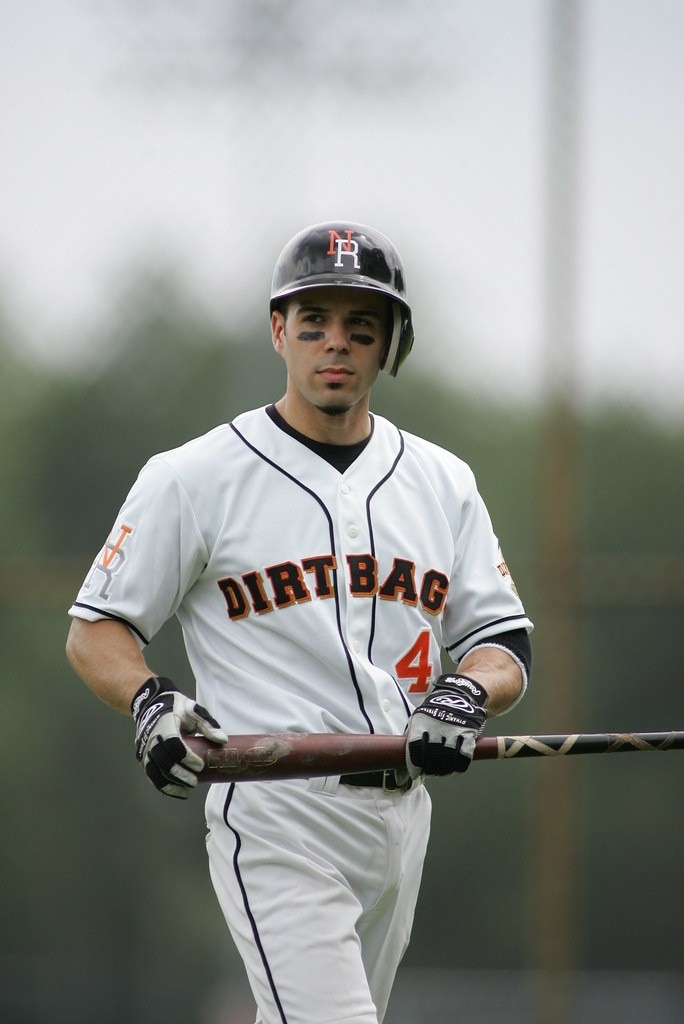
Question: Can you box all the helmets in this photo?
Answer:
[269,218,415,378]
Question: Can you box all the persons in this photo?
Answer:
[67,224,535,1024]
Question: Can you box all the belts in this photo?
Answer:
[339,767,413,792]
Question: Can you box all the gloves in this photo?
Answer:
[404,673,488,780]
[131,676,228,801]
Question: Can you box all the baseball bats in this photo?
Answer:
[170,726,684,785]
